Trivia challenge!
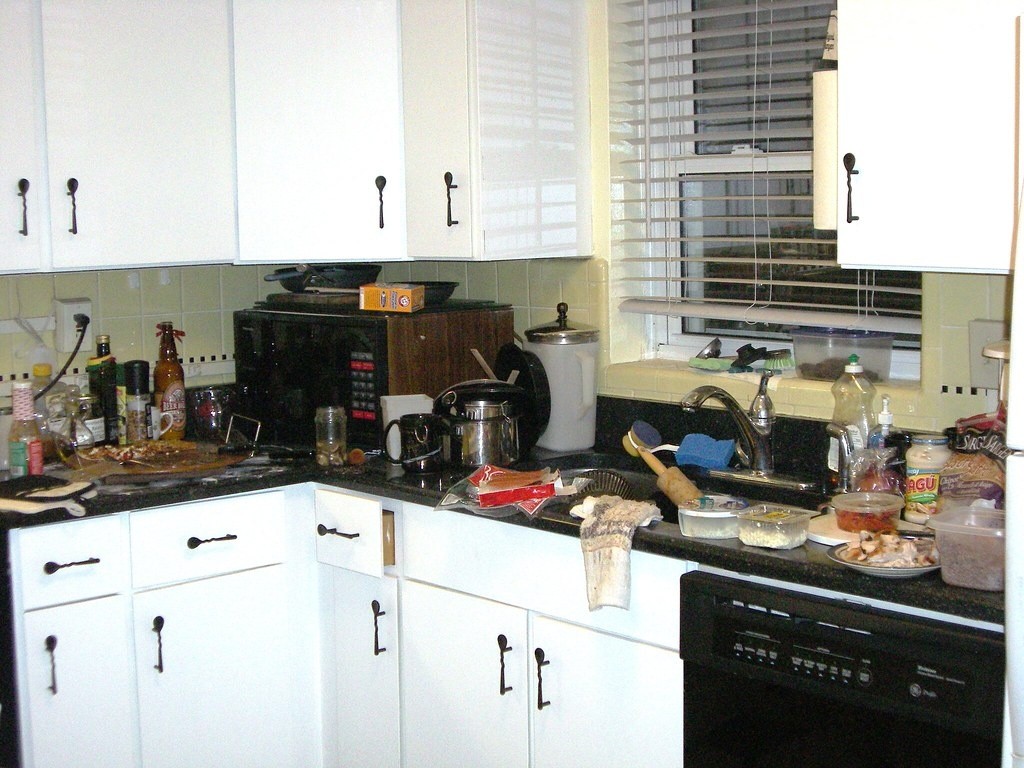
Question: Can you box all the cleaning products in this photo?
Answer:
[828,353,879,485]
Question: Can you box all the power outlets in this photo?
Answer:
[54,296,94,353]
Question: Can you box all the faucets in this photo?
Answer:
[680,369,776,469]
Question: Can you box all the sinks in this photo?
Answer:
[537,455,681,506]
[645,484,828,531]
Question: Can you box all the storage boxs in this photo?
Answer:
[361,283,425,313]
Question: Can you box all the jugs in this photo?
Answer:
[520,301,599,451]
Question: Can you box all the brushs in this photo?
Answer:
[623,420,679,456]
[717,349,794,372]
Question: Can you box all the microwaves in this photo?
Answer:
[232,303,513,459]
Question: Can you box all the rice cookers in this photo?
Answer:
[434,381,525,471]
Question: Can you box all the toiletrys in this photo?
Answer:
[867,393,902,449]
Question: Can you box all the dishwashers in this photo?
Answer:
[679,567,1005,768]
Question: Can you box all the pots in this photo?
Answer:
[274,263,381,293]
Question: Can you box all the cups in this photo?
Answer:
[380,412,436,472]
[151,407,173,440]
[316,407,347,465]
[193,389,229,442]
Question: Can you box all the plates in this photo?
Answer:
[826,542,942,576]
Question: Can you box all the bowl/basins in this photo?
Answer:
[732,504,821,549]
[678,504,747,539]
[926,505,1005,592]
[790,330,894,384]
[833,492,904,533]
[392,280,458,302]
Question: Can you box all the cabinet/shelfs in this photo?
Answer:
[0,0,595,276]
[8,481,699,768]
[837,1,1023,274]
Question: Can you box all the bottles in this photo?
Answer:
[59,384,94,453]
[827,354,876,498]
[32,363,70,439]
[89,335,118,444]
[125,360,150,445]
[9,381,43,478]
[154,321,187,439]
[867,393,901,452]
